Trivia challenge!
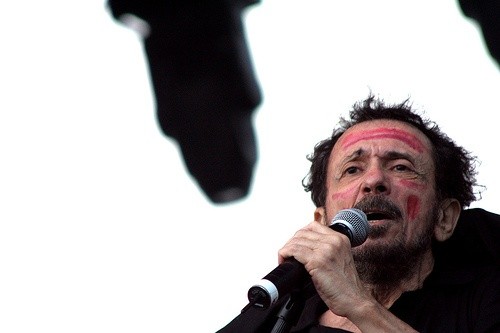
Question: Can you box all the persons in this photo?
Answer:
[215,94,499,332]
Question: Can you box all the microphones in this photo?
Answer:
[239,206,371,316]
